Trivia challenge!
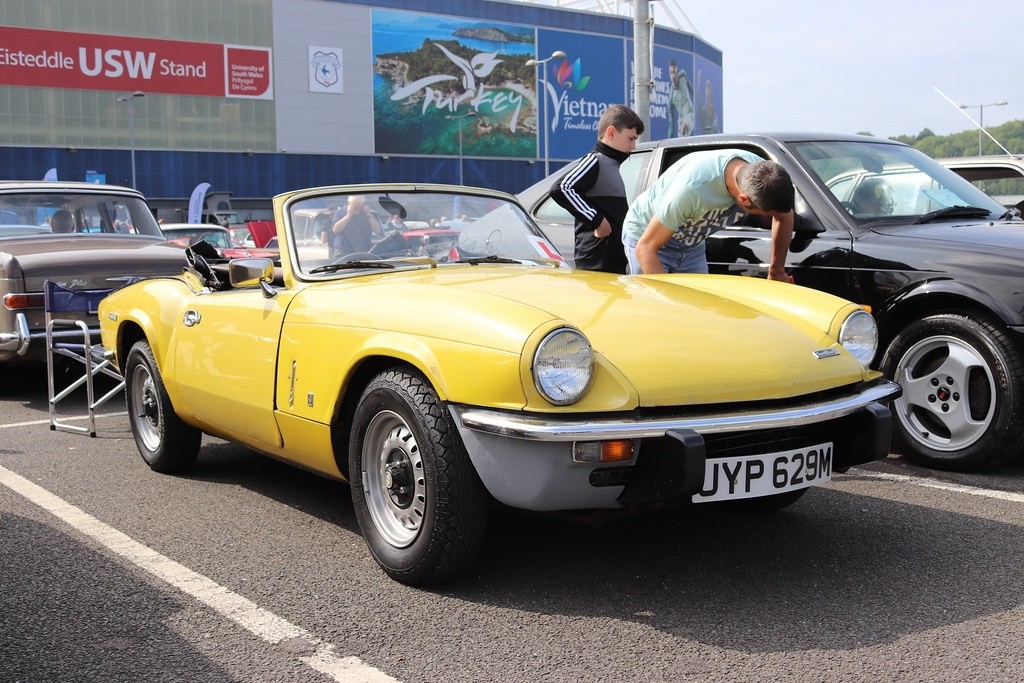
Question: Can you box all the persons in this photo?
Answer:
[623,149,795,284]
[333,196,382,262]
[549,105,644,276]
[318,204,339,258]
[430,219,436,227]
[111,201,130,234]
[455,214,466,222]
[41,217,51,226]
[222,216,229,227]
[696,70,720,134]
[392,214,406,228]
[667,60,695,139]
[50,210,74,233]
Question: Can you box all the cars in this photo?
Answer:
[127,224,281,262]
[0,181,189,362]
[457,131,1024,473]
[96,182,903,581]
[820,155,1024,216]
[242,207,461,259]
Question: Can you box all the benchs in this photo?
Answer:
[207,262,319,291]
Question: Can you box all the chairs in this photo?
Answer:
[0,210,20,228]
[852,178,896,219]
[42,277,143,437]
[50,209,76,234]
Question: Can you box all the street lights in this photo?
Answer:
[445,111,477,185]
[959,101,1006,192]
[117,90,145,192]
[526,50,568,179]
